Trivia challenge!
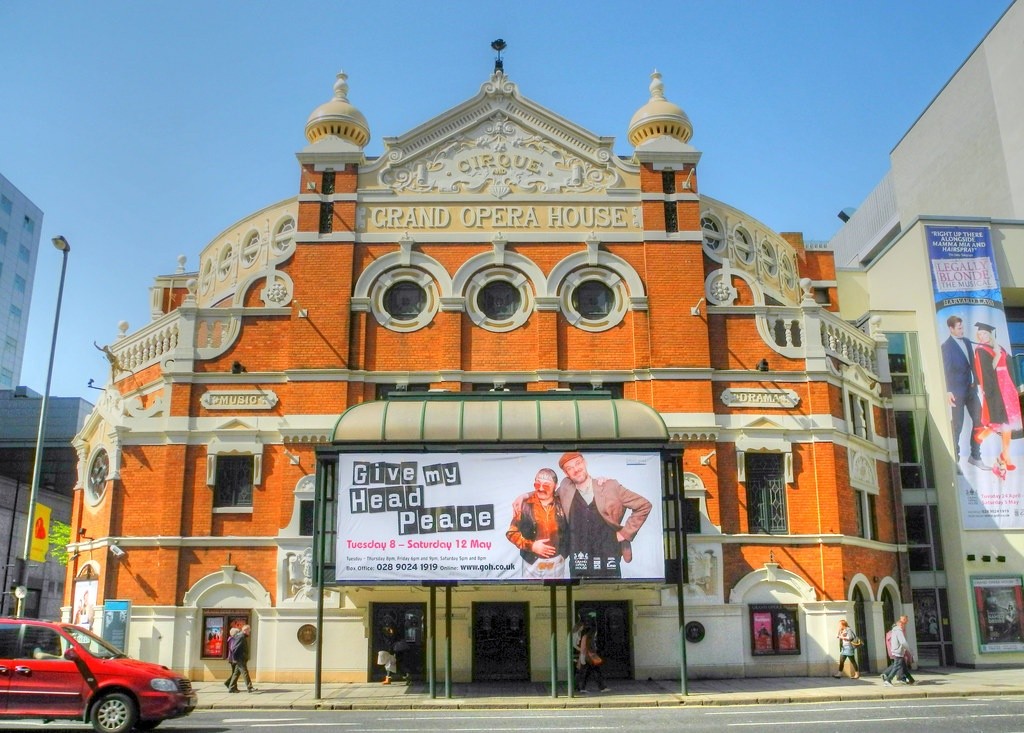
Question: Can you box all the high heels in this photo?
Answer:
[1000,452,1016,470]
[974,426,985,443]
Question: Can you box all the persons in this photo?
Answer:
[880,623,909,685]
[973,322,1022,471]
[573,609,593,695]
[506,468,609,579]
[833,620,860,679]
[897,615,914,685]
[224,628,242,694]
[512,452,653,578]
[31,629,63,660]
[74,590,95,625]
[378,615,412,685]
[576,617,611,694]
[228,625,258,693]
[928,617,938,635]
[941,316,993,475]
[884,620,920,688]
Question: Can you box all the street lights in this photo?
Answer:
[13,233,73,619]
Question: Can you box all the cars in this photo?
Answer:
[1,615,198,733]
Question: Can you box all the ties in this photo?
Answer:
[957,337,1002,353]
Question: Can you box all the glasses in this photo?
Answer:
[534,482,554,490]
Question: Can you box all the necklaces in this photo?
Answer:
[540,502,556,523]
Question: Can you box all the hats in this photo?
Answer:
[974,321,997,338]
[559,452,582,468]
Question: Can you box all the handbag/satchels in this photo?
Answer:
[851,636,864,648]
[590,653,602,665]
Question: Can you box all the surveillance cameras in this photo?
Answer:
[110,545,125,557]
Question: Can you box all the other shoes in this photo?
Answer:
[968,455,992,470]
[882,674,886,681]
[580,689,587,692]
[601,687,611,692]
[897,680,906,684]
[956,463,963,475]
[248,687,258,692]
[911,680,920,685]
[229,689,239,692]
[885,679,893,686]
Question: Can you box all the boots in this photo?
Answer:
[403,672,411,685]
[833,670,842,678]
[382,675,392,684]
[851,670,860,679]
